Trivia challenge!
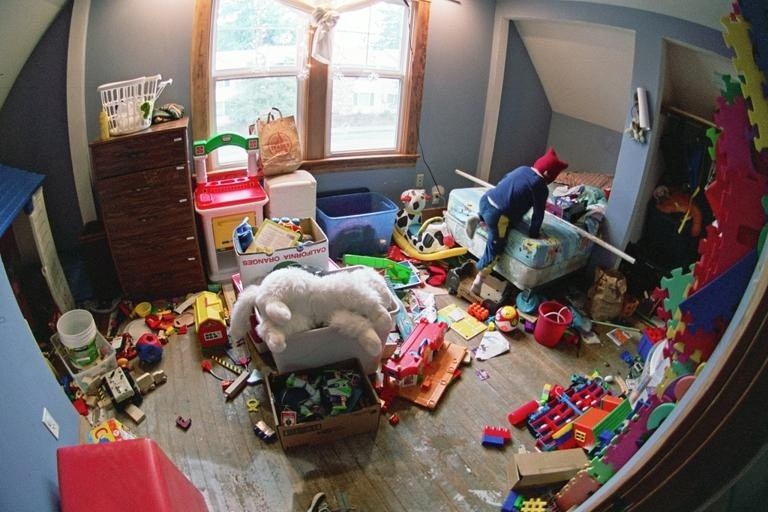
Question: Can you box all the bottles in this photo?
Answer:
[98,112,110,140]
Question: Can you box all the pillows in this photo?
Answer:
[554,168,613,193]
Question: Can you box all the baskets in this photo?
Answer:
[97,75,172,136]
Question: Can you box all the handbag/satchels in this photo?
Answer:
[249,108,302,175]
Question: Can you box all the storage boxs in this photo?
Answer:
[231,192,401,451]
[49,327,118,398]
[507,447,590,491]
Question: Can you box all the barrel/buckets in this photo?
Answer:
[534,301,573,347]
[57,309,99,366]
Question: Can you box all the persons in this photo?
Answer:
[465,148,568,296]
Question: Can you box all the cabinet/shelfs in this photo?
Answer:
[87,117,207,302]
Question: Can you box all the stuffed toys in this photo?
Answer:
[227,267,393,355]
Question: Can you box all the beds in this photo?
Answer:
[442,180,610,292]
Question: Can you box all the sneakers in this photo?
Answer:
[470,283,480,297]
[467,217,476,239]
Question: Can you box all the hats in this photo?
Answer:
[533,149,568,185]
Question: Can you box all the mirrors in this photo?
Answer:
[0,1,768,512]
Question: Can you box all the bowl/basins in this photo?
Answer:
[136,301,151,318]
